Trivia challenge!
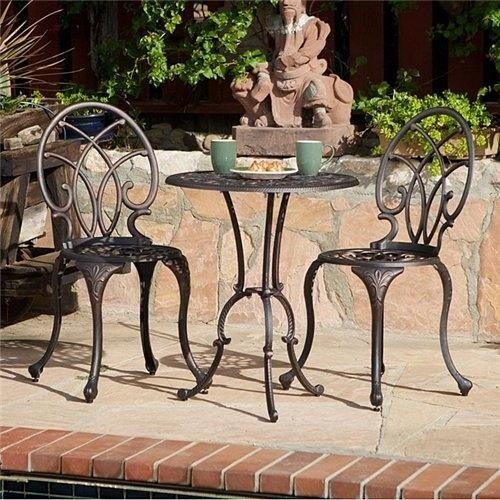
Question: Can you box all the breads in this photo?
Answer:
[248,160,283,171]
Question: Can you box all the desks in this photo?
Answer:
[165,168,359,421]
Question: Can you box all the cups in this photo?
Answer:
[296,140,334,175]
[209,139,237,174]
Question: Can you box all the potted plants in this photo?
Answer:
[56,92,117,142]
[350,81,499,176]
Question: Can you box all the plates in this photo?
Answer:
[229,166,295,179]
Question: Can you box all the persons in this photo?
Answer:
[229,0,355,127]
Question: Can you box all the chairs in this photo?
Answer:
[28,101,212,403]
[280,106,472,410]
